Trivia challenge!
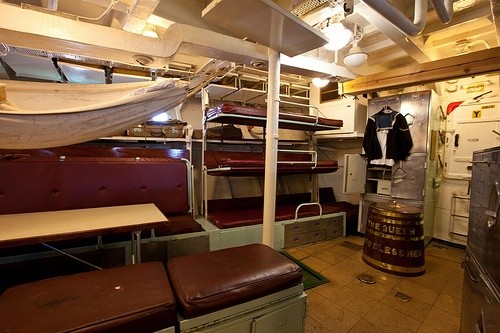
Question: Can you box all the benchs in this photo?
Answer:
[0,257,177,333]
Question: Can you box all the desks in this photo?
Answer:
[0,199,169,266]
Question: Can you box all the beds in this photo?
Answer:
[200,84,345,248]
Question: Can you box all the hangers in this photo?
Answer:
[371,98,401,117]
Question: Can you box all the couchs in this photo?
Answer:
[0,157,205,294]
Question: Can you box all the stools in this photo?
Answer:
[164,243,309,333]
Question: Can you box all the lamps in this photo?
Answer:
[312,13,369,88]
